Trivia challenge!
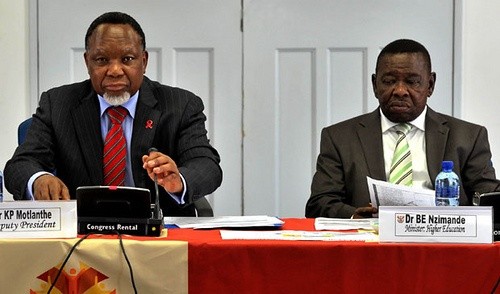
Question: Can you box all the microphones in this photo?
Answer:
[149,148,162,219]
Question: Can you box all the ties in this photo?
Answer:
[104,106,127,186]
[388,122,414,186]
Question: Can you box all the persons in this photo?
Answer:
[3,11,223,217]
[305,39,500,219]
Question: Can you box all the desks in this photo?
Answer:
[0,217,500,294]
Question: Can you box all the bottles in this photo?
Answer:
[435,161,460,207]
